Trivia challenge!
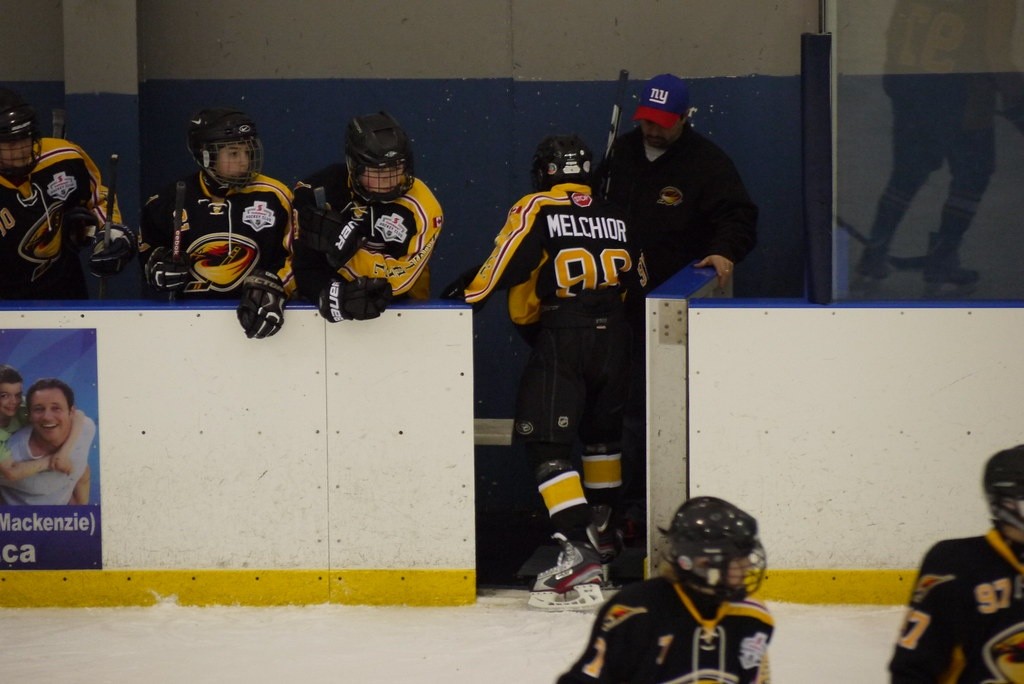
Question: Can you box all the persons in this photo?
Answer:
[0,86,135,300]
[849,0,1024,302]
[137,105,300,339]
[566,75,757,548]
[292,109,445,324]
[0,362,96,505]
[887,443,1024,684]
[556,495,773,684]
[439,128,645,612]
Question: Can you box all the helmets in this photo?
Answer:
[986,445,1023,561]
[532,135,592,190]
[0,91,43,176]
[345,110,412,204]
[671,498,765,603]
[185,106,263,189]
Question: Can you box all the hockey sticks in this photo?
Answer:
[598,69,630,203]
[166,181,187,303]
[98,154,119,301]
[313,186,341,268]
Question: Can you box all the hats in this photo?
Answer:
[633,73,688,128]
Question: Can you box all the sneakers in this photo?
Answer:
[924,232,980,298]
[528,532,604,611]
[596,502,627,584]
[854,241,889,289]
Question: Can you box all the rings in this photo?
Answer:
[725,270,729,271]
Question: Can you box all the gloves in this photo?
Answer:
[140,247,187,291]
[442,278,472,307]
[304,206,356,252]
[88,225,134,278]
[314,274,392,322]
[235,269,286,338]
